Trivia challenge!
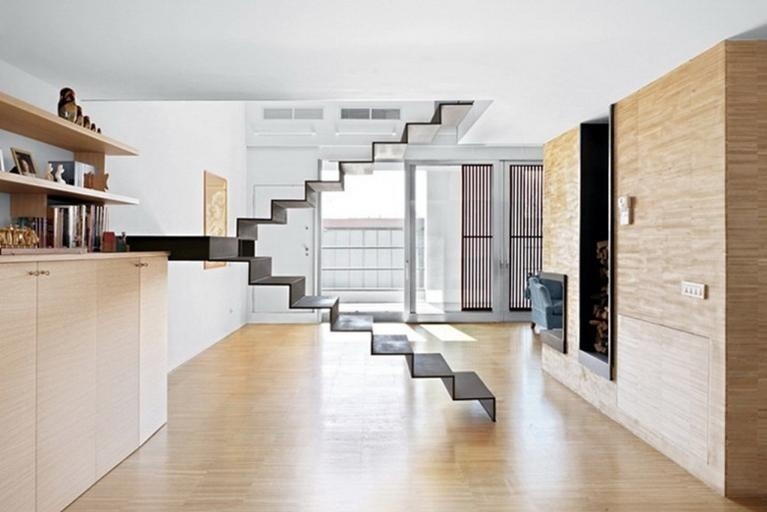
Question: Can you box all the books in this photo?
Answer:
[47,158,96,189]
[17,202,110,250]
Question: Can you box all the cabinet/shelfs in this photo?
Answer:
[0,255,97,511]
[97,255,168,486]
[0,92,142,253]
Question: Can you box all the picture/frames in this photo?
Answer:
[203,169,228,270]
